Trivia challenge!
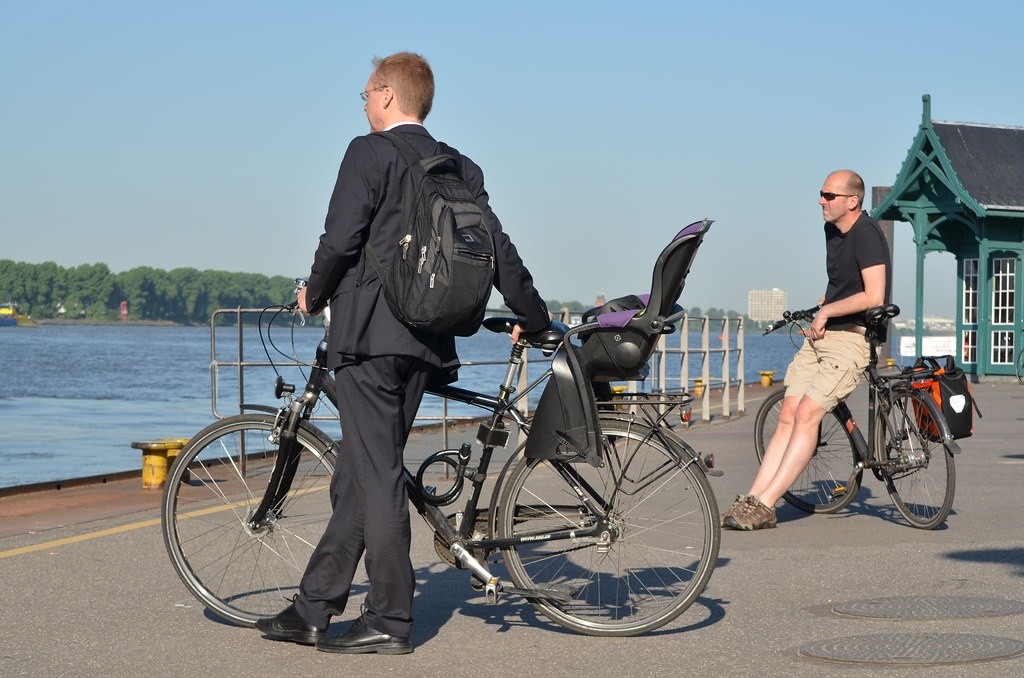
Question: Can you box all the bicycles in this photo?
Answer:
[1016,329,1024,385]
[754,304,962,529]
[161,219,726,636]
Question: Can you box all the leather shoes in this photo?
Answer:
[316,604,413,655]
[254,593,330,644]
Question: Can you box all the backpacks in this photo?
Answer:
[354,131,496,338]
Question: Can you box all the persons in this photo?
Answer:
[719,171,892,530]
[254,52,553,654]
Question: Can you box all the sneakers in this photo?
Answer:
[720,494,777,531]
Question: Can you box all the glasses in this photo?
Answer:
[820,190,860,201]
[360,85,388,101]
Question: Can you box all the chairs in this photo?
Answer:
[523,216,714,468]
